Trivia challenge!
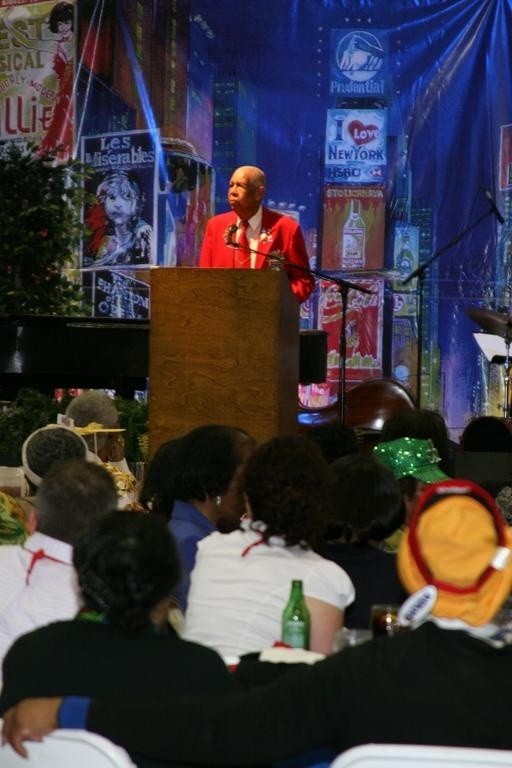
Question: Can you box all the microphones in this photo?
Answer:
[225,224,238,245]
[485,191,504,224]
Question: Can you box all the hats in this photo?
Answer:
[374,437,449,484]
[21,425,102,484]
[399,479,512,625]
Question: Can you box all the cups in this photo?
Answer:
[371,603,403,638]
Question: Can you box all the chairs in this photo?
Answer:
[328,739,512,766]
[1,725,138,768]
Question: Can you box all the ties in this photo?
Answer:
[233,221,251,269]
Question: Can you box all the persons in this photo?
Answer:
[0,381,511,766]
[92,167,152,265]
[43,0,75,42]
[199,166,315,304]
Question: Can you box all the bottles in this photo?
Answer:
[278,577,313,652]
[339,196,367,269]
[395,229,415,293]
